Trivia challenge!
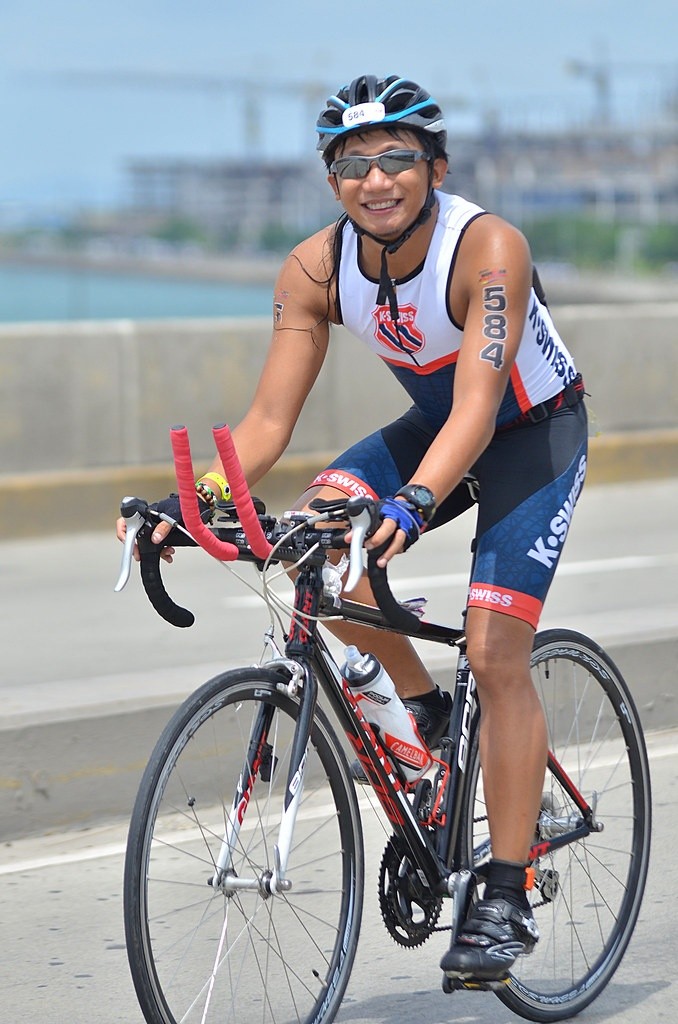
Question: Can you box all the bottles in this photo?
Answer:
[339,646,431,784]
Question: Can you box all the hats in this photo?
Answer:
[315,76,446,153]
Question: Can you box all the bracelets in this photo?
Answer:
[193,472,231,512]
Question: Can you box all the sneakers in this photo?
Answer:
[442,893,537,980]
[350,689,456,785]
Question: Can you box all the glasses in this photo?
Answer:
[332,150,429,176]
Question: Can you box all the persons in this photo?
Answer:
[116,75,590,983]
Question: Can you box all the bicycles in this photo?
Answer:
[117,425,653,1023]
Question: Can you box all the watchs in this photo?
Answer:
[393,483,436,531]
